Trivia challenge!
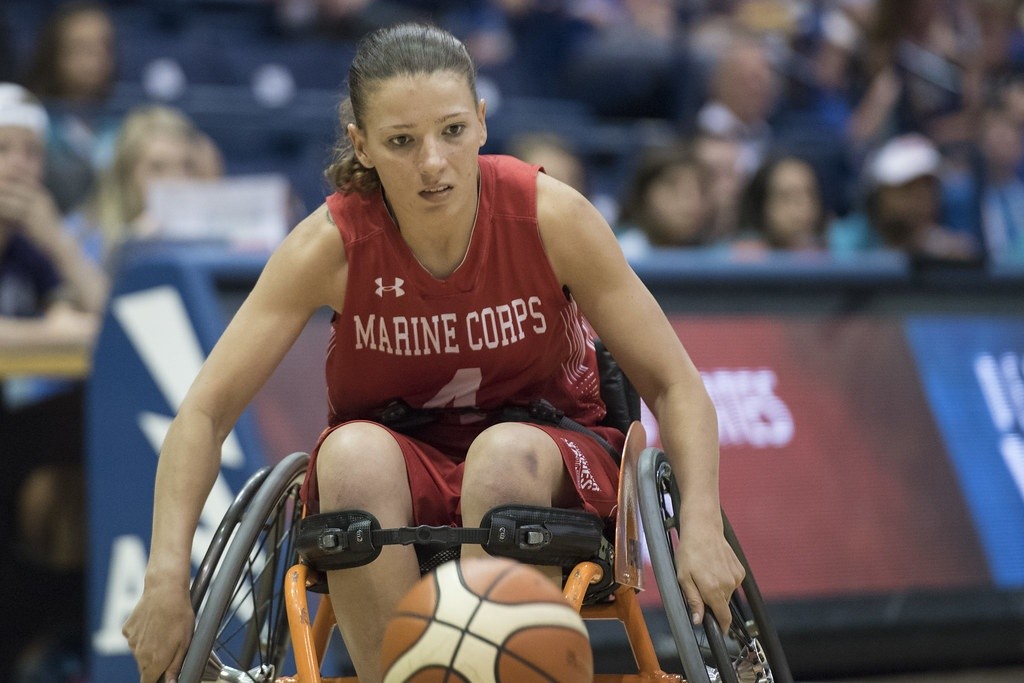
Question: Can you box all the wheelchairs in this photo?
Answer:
[167,335,799,683]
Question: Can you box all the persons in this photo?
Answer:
[0,79,113,649]
[33,0,225,243]
[122,24,746,683]
[505,0,1024,270]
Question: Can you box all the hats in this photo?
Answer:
[0,79,49,137]
[864,130,938,183]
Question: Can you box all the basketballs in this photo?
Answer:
[377,555,596,683]
